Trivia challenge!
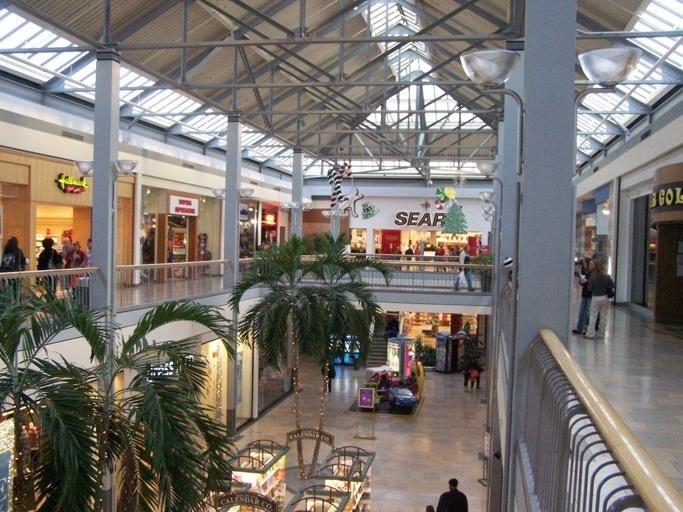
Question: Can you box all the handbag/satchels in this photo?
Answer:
[607,287,616,298]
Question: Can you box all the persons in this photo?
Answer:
[425,504,434,511]
[453,244,476,291]
[321,364,336,393]
[476,364,483,387]
[571,259,597,334]
[0,235,29,302]
[60,237,72,253]
[37,237,62,305]
[503,255,513,280]
[83,237,91,266]
[462,367,469,391]
[62,239,85,300]
[469,367,479,388]
[436,477,468,512]
[395,244,464,273]
[582,263,615,339]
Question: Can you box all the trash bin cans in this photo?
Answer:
[76,277,90,310]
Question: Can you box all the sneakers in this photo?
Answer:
[570,328,605,339]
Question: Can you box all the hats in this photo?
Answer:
[502,256,513,269]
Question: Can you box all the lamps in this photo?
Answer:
[322,210,350,220]
[460,47,639,91]
[209,187,255,200]
[74,159,136,177]
[476,160,497,221]
[281,200,314,212]
